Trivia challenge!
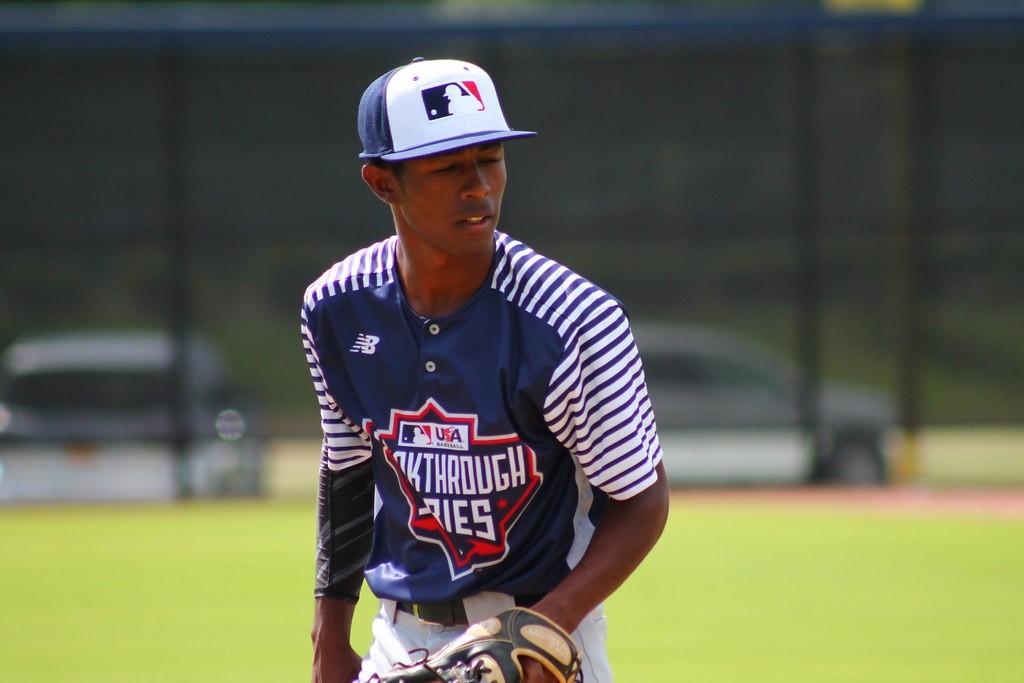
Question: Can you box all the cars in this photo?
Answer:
[1,332,268,501]
[631,317,898,489]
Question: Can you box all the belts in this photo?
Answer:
[397,591,551,628]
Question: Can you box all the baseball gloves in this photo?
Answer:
[361,607,586,683]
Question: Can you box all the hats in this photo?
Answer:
[358,57,538,161]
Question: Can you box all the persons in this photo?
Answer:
[299,56,671,682]
